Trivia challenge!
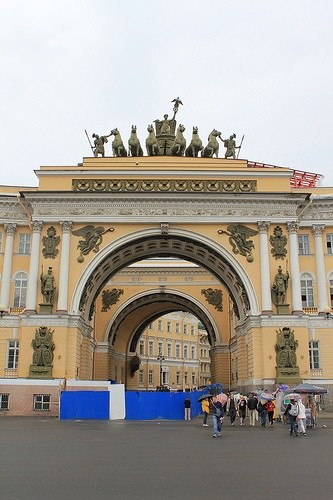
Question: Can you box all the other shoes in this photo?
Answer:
[203,424,209,426]
[288,431,306,437]
[217,432,221,437]
[213,434,217,438]
[231,420,276,427]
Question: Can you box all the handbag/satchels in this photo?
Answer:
[217,409,221,416]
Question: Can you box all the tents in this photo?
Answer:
[289,383,327,395]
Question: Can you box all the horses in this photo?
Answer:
[146,124,159,156]
[170,123,186,156]
[186,126,204,158]
[128,124,144,157]
[111,128,127,157]
[201,128,223,158]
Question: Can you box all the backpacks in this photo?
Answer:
[288,403,298,416]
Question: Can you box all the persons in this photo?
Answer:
[42,228,60,257]
[41,269,56,304]
[271,229,287,257]
[222,135,241,159]
[223,392,280,426]
[209,396,224,438]
[201,398,210,427]
[183,398,191,421]
[276,327,299,368]
[32,326,53,367]
[159,114,176,134]
[91,134,109,157]
[283,398,307,437]
[273,267,290,303]
[309,396,318,422]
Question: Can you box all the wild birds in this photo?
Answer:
[171,96,183,105]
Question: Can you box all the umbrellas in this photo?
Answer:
[198,394,212,402]
[258,393,275,400]
[216,394,227,407]
[285,394,302,399]
